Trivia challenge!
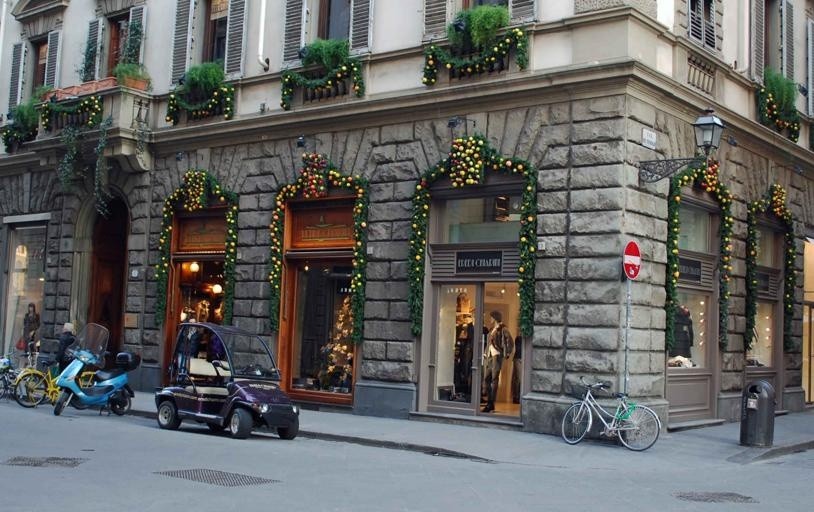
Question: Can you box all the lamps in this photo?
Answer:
[639,105,726,187]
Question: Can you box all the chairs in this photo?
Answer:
[214,355,230,383]
[179,353,227,398]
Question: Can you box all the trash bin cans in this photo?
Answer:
[739,379,777,447]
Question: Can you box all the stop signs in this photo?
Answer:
[623,238,641,280]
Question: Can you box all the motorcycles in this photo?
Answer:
[53,321,141,414]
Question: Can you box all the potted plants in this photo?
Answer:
[33,58,152,100]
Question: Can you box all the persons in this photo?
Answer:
[22,302,41,368]
[511,335,523,404]
[481,310,514,413]
[56,322,77,376]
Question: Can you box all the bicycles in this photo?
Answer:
[558,374,661,452]
[0,349,99,408]
